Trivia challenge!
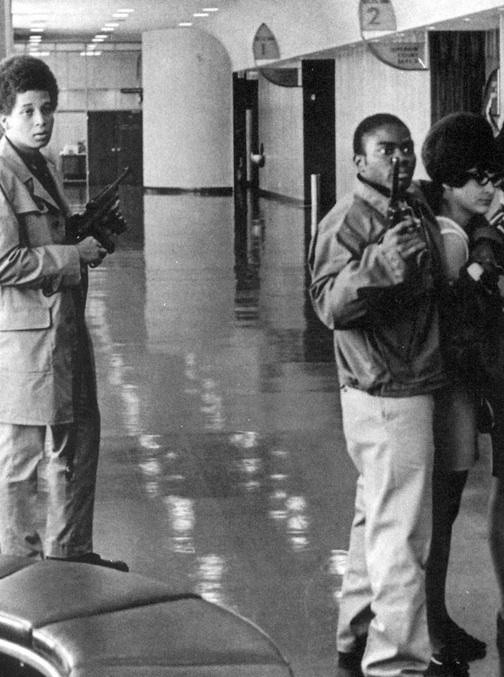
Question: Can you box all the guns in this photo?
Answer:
[63,168,130,267]
[382,157,431,267]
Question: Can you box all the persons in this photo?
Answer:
[0,53,132,574]
[410,112,502,657]
[307,111,463,674]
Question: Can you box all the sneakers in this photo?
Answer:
[75,552,129,573]
[338,633,470,677]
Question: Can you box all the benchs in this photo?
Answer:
[0,554,295,677]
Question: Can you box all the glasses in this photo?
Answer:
[464,170,503,187]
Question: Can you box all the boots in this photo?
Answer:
[424,470,488,662]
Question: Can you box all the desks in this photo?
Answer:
[60,153,87,187]
